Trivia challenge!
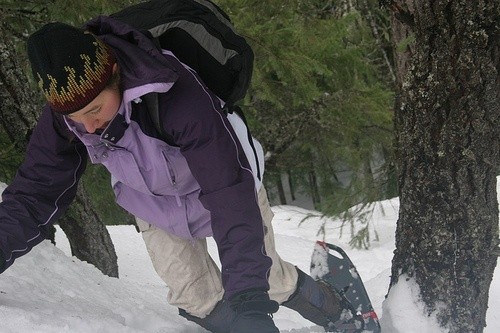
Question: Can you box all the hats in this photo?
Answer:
[27,22,114,115]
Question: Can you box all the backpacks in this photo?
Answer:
[54,0,254,148]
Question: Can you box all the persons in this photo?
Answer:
[0,0,365,333]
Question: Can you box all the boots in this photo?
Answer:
[178,291,232,333]
[280,266,364,333]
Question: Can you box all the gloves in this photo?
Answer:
[230,290,280,333]
